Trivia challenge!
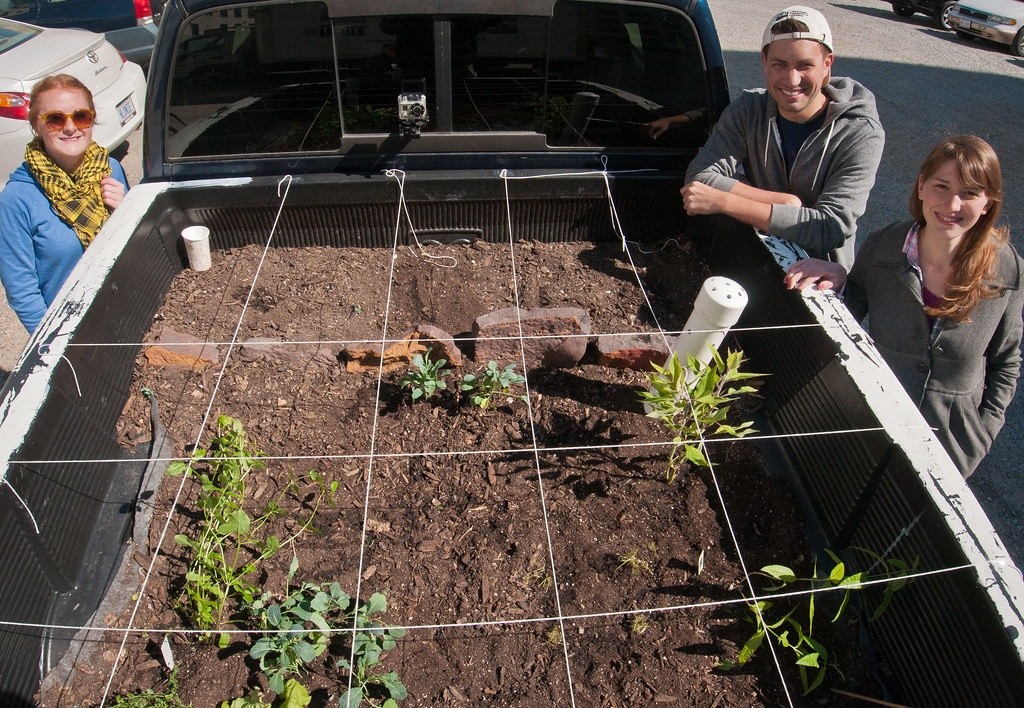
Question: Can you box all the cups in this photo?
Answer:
[182,226,211,271]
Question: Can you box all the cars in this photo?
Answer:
[948,0,1024,57]
[0,16,147,193]
[882,0,958,31]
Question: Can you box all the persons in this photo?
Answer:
[679,5,882,278]
[0,74,129,340]
[781,134,1024,481]
[644,105,708,141]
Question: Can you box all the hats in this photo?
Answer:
[761,6,833,87]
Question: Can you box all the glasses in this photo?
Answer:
[36,108,95,132]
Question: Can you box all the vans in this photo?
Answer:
[0,0,169,64]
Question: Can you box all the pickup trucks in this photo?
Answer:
[1,1,1024,701]
